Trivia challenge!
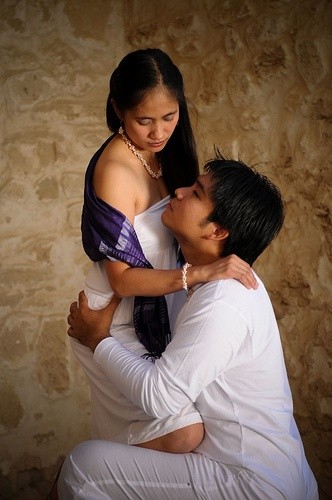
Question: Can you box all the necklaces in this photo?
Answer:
[118,127,163,178]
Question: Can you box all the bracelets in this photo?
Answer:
[181,262,196,297]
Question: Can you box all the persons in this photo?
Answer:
[69,48,260,454]
[58,145,318,500]
